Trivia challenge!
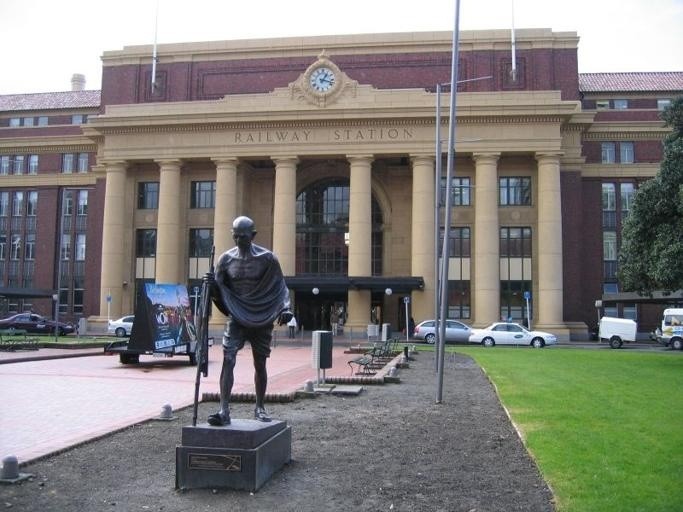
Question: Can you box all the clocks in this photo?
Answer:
[302,59,342,99]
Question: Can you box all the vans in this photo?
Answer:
[659,307,682,352]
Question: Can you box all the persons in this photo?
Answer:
[202,215,294,426]
[315,306,345,337]
[286,315,297,338]
[406,317,416,339]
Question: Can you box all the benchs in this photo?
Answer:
[347,336,401,377]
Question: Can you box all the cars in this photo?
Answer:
[410,316,558,348]
[0,312,75,338]
[106,313,136,337]
